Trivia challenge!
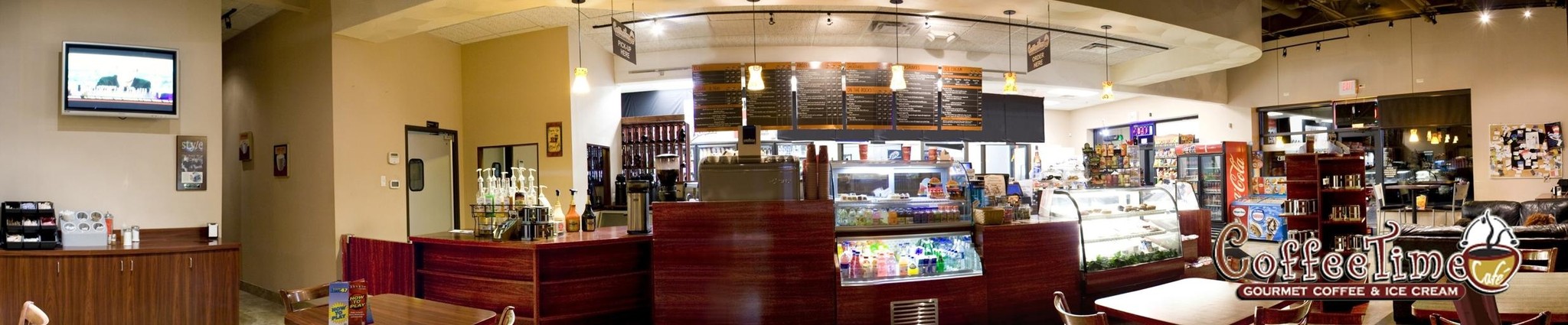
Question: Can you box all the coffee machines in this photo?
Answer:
[655,153,680,201]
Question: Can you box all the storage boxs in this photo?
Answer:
[1182,238,1198,262]
[1183,263,1218,279]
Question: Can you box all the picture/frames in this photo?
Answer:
[272,143,290,178]
[238,131,253,161]
[545,121,563,157]
[175,133,207,193]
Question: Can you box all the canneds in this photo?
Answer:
[911,205,961,224]
[1095,143,1131,187]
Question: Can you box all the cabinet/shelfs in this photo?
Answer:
[626,140,816,186]
[1082,139,1143,188]
[830,155,989,324]
[1038,187,1185,307]
[1153,176,1212,261]
[0,236,241,324]
[1276,150,1371,324]
[622,113,690,202]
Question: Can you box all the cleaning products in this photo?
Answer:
[473,161,598,237]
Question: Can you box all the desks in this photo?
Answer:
[1411,270,1568,324]
[1093,277,1300,325]
[283,292,497,325]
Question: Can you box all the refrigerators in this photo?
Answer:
[1176,141,1285,241]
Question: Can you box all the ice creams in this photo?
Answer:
[1265,217,1280,240]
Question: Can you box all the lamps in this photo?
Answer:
[570,1,591,96]
[747,1,766,94]
[1101,25,1113,102]
[889,0,909,91]
[1002,11,1018,94]
[1409,128,1459,145]
[1262,26,1350,58]
[645,10,933,43]
[1387,20,1393,28]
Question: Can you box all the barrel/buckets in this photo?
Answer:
[1181,233,1199,264]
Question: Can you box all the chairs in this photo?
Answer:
[1253,298,1312,325]
[497,305,517,325]
[1053,289,1107,324]
[19,300,50,325]
[1226,254,1301,284]
[1429,308,1552,324]
[1432,180,1471,225]
[1516,247,1558,272]
[278,279,343,313]
[1373,183,1406,235]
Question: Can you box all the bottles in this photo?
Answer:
[837,237,977,278]
[1034,146,1041,180]
[105,213,140,246]
[839,206,960,226]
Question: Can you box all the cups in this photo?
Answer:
[240,142,248,154]
[902,145,911,161]
[859,143,868,159]
[276,155,286,171]
[928,147,937,162]
[803,144,830,199]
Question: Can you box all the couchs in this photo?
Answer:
[1391,199,1568,271]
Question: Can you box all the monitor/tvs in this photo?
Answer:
[61,41,180,119]
